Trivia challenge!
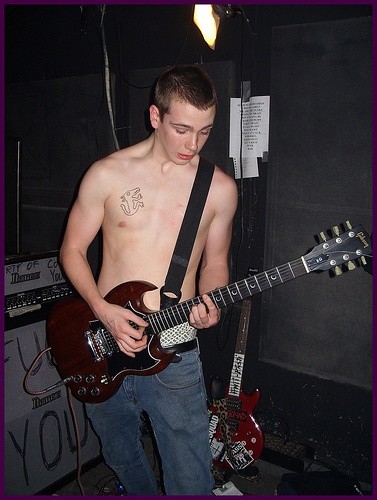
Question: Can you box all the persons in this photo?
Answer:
[60,64,239,497]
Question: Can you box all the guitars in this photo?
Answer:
[209,265,268,474]
[45,218,372,405]
